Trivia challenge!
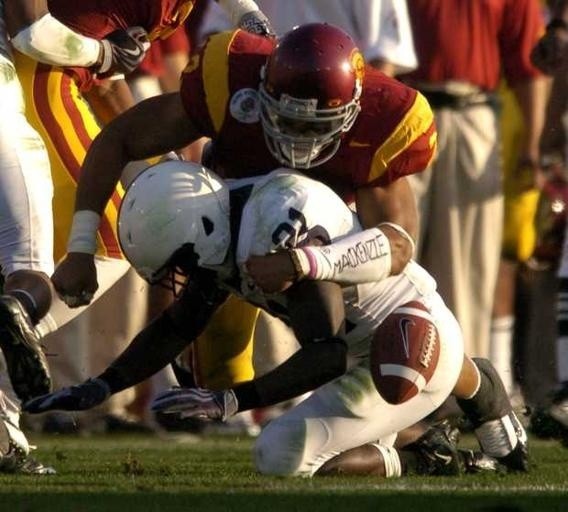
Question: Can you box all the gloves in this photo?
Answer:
[94,24,152,81]
[21,375,112,414]
[150,385,240,422]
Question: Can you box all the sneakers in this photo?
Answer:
[410,407,501,476]
[509,380,567,443]
[0,291,52,400]
[0,442,58,475]
[452,352,529,468]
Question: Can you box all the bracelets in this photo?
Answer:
[66,211,101,255]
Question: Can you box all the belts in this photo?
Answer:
[419,84,490,111]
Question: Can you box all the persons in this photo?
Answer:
[23,158,497,480]
[2,0,275,475]
[51,21,529,471]
[207,2,567,442]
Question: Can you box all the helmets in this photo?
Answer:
[116,158,232,286]
[262,19,367,121]
